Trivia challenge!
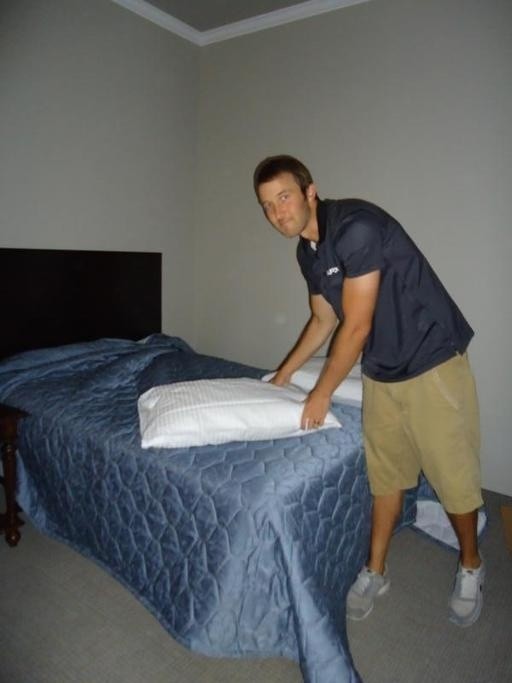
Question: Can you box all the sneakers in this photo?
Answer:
[345,561,391,622]
[448,549,486,629]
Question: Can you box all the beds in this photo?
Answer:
[0,246,428,658]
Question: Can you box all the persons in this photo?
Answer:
[254,155,486,626]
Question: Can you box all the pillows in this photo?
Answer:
[134,350,369,449]
